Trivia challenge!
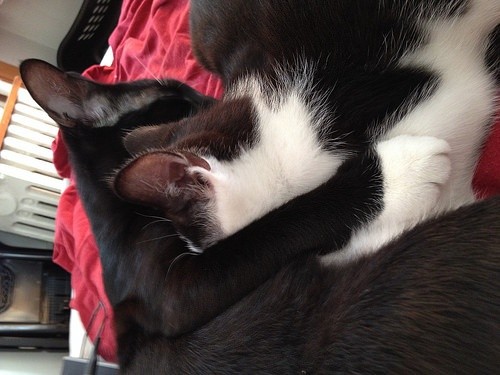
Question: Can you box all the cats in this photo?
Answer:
[110,0,500,266]
[18,57,500,375]
